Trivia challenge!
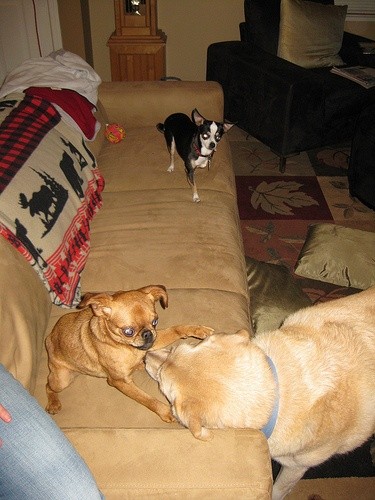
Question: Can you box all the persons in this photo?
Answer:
[0,364,105,500]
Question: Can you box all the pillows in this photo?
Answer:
[245,255,313,336]
[293,221,375,289]
[277,0,349,69]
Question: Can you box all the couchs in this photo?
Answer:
[206,0,375,168]
[0,81,273,500]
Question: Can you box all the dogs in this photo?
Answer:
[155,107,236,202]
[45,284,215,426]
[142,284,375,500]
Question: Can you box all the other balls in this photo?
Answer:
[105,123,125,143]
